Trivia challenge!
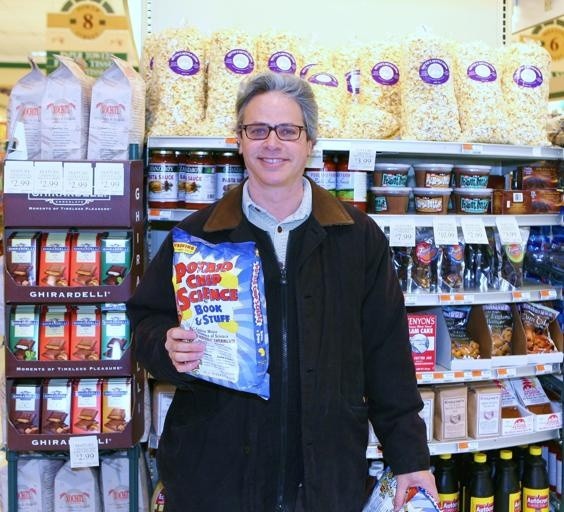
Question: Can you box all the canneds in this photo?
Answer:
[148,147,248,209]
[306,151,367,214]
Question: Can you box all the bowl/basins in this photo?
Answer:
[368,161,494,214]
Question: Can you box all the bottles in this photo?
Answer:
[368,441,548,512]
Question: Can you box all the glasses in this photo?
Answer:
[238,123,307,142]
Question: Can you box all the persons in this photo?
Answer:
[125,72,438,512]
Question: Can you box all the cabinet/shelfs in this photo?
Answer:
[147,133,564,512]
[0,158,147,512]
